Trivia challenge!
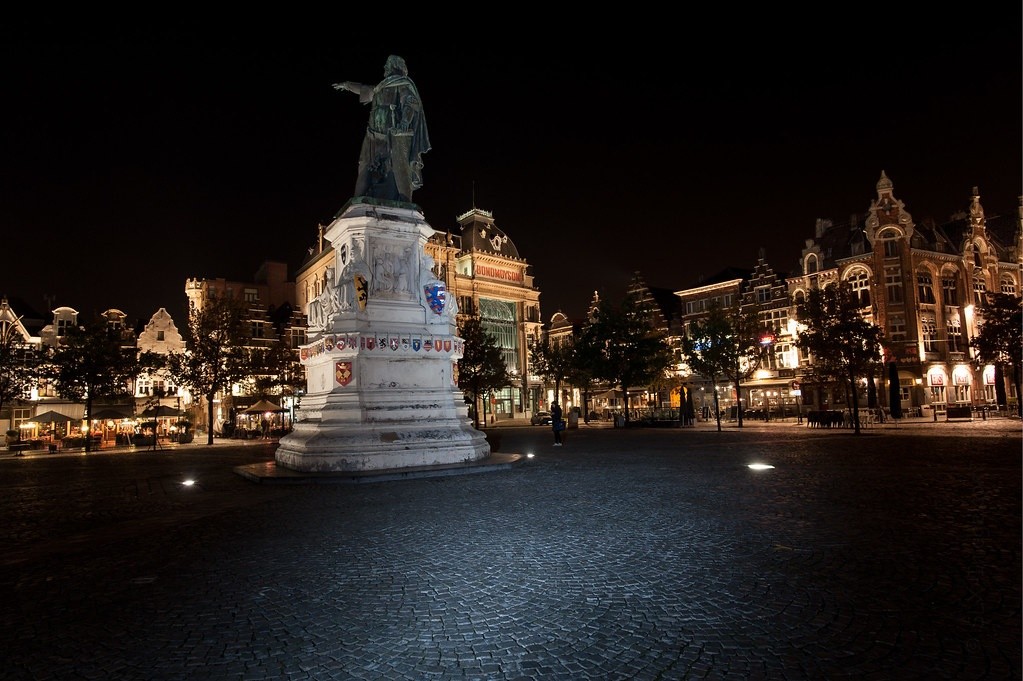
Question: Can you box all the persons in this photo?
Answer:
[332,55,429,204]
[262,418,270,439]
[551,401,563,446]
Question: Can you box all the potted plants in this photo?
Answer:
[178,421,193,443]
[141,422,158,445]
[247,431,253,439]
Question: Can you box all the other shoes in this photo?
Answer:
[553,443,562,447]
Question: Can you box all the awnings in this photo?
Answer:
[738,378,796,387]
[681,374,741,382]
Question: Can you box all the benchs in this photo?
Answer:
[903,407,919,418]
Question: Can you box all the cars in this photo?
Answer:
[530,412,552,426]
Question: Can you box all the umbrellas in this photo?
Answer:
[28,410,74,442]
[83,409,130,420]
[137,405,187,417]
[240,400,289,413]
[593,390,624,406]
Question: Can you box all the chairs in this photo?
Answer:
[807,411,878,430]
